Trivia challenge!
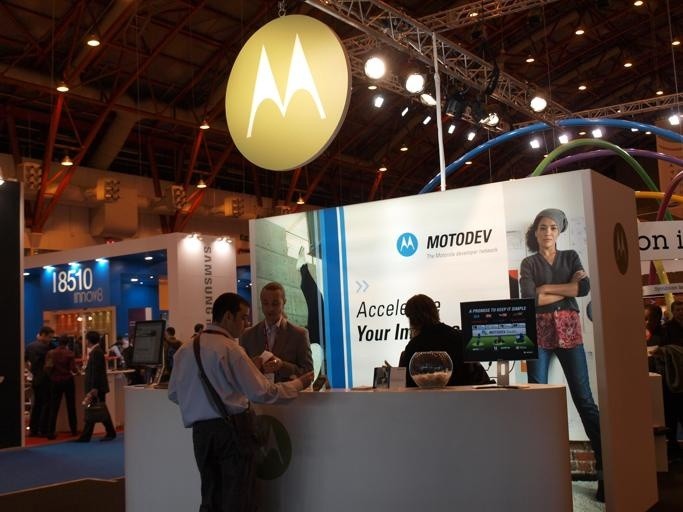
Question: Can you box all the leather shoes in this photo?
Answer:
[100,433,117,442]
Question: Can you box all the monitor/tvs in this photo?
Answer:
[460,297,538,389]
[130,319,165,389]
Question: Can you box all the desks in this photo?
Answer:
[124,383,573,512]
[57,370,135,433]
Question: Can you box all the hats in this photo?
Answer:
[536,208,569,236]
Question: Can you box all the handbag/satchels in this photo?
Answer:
[191,333,261,456]
[83,394,108,423]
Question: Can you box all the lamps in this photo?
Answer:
[199,115,210,130]
[465,161,473,166]
[367,84,378,90]
[624,59,632,67]
[559,131,572,144]
[525,54,534,62]
[530,137,543,148]
[87,32,100,46]
[668,113,681,126]
[196,174,207,188]
[471,107,489,124]
[399,143,409,151]
[592,126,606,138]
[378,165,387,171]
[526,56,535,62]
[400,103,410,120]
[575,26,584,35]
[145,256,153,261]
[420,115,432,126]
[420,113,432,127]
[61,155,73,165]
[445,96,464,117]
[57,83,69,92]
[672,38,680,45]
[296,193,305,205]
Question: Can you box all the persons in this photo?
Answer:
[44,334,82,440]
[73,332,117,443]
[74,336,82,355]
[190,323,204,339]
[662,301,683,347]
[661,311,670,322]
[238,282,314,382]
[643,304,683,454]
[25,326,55,437]
[164,327,183,371]
[168,292,314,512]
[107,341,124,365]
[398,294,491,387]
[520,209,606,503]
